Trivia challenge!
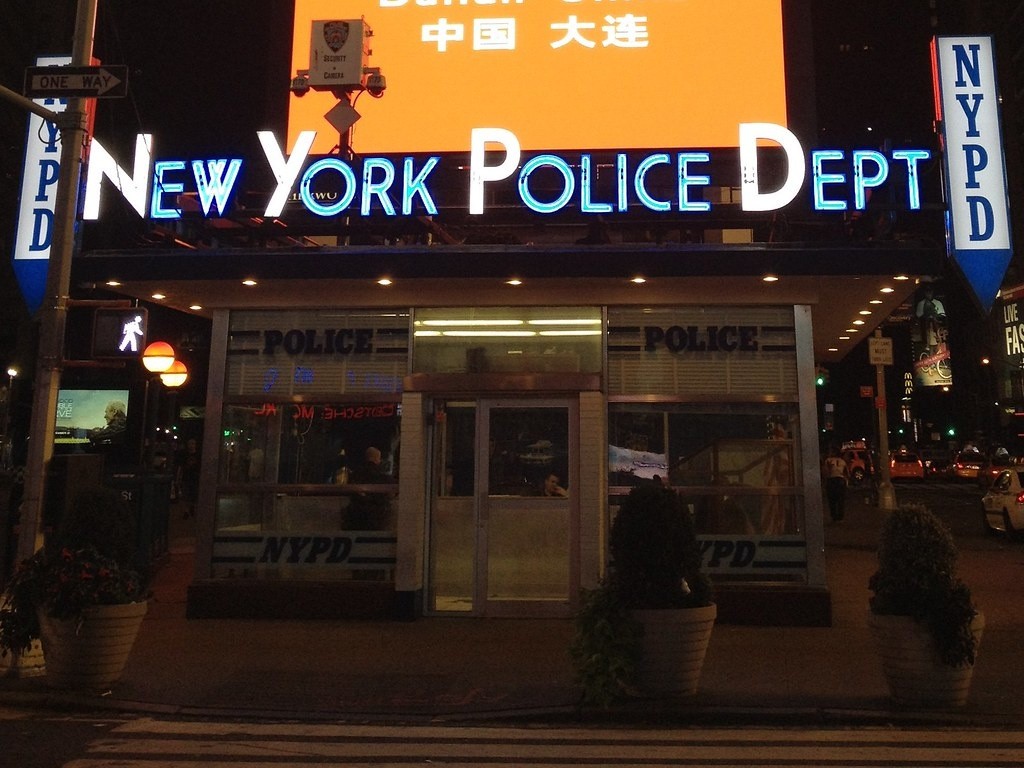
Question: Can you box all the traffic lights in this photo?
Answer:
[92,306,146,359]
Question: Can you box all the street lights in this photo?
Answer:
[141,340,175,598]
[158,360,188,559]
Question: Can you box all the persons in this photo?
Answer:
[542,471,568,498]
[353,447,383,484]
[822,447,850,523]
[176,438,201,521]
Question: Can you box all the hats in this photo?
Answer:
[154,449,167,456]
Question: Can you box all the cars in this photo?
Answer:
[979,459,1016,491]
[976,465,1024,540]
[952,449,987,483]
[887,452,924,484]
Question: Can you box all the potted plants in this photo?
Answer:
[0,522,156,691]
[866,502,986,717]
[575,481,717,704]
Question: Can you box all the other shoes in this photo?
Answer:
[155,545,168,557]
[835,520,844,523]
[183,512,189,520]
[190,511,194,517]
[171,498,179,502]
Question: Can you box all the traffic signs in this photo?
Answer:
[24,64,129,99]
